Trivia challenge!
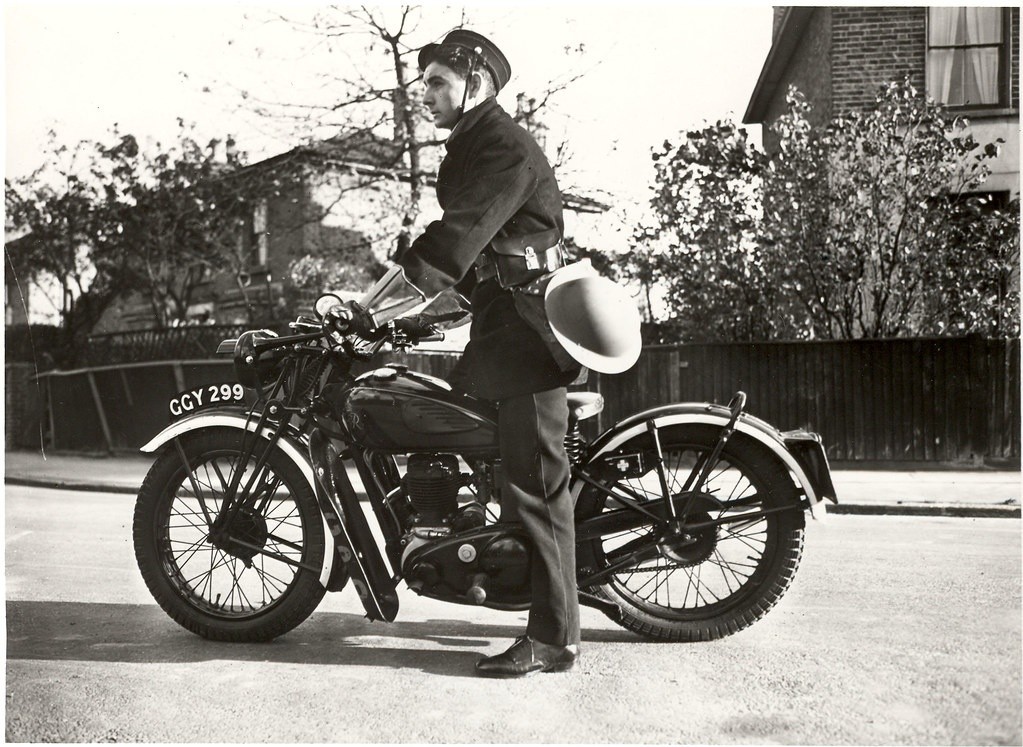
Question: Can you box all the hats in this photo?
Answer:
[544,263,642,374]
[417,29,511,97]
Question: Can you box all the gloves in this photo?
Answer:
[323,264,426,343]
[393,285,472,345]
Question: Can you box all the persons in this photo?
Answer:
[326,28,579,682]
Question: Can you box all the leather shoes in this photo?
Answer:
[475,633,580,678]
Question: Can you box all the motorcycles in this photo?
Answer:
[132,297,838,646]
